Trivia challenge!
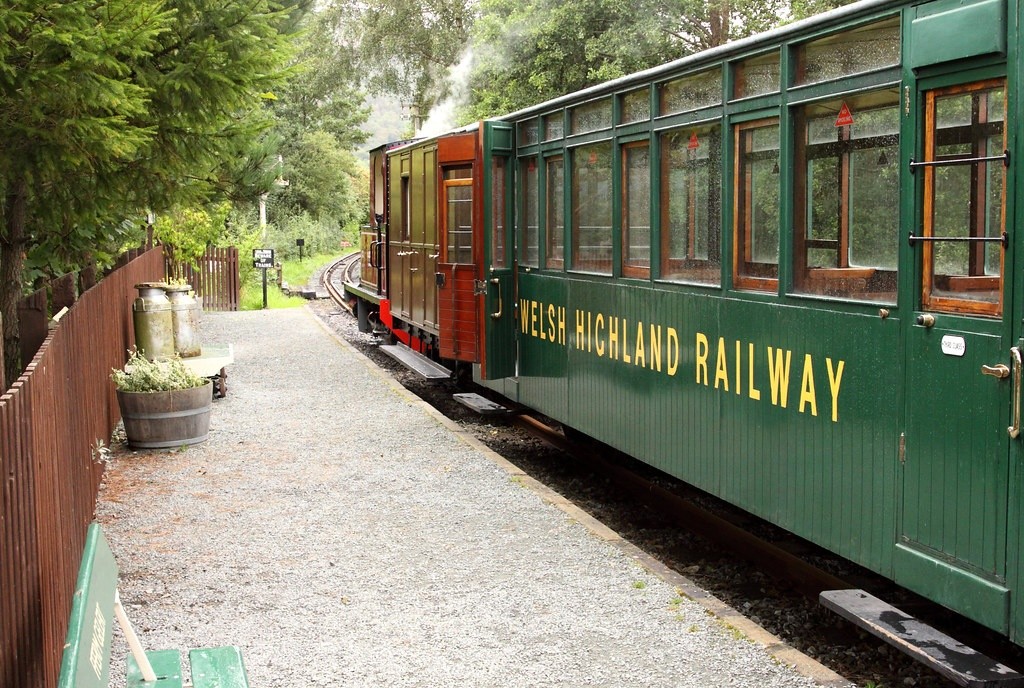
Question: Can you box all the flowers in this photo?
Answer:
[109,344,203,393]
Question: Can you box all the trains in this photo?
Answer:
[340,0,1023,688]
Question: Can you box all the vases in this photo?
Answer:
[114,379,213,448]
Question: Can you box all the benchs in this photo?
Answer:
[808,268,1001,304]
[57,523,249,688]
